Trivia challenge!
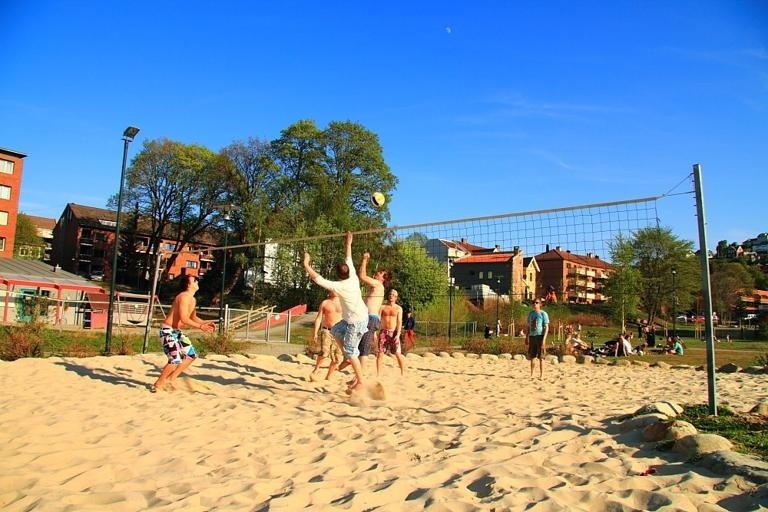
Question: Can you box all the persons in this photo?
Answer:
[527,298,550,381]
[304,232,415,389]
[520,328,524,337]
[154,275,216,390]
[563,321,685,363]
[495,318,504,338]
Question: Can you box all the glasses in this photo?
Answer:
[532,302,541,304]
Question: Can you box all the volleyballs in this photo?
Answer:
[370,193,386,207]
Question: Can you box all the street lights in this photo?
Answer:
[448,259,454,344]
[210,199,242,336]
[669,268,677,338]
[100,124,138,357]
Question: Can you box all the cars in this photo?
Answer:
[88,270,104,282]
[675,313,756,324]
